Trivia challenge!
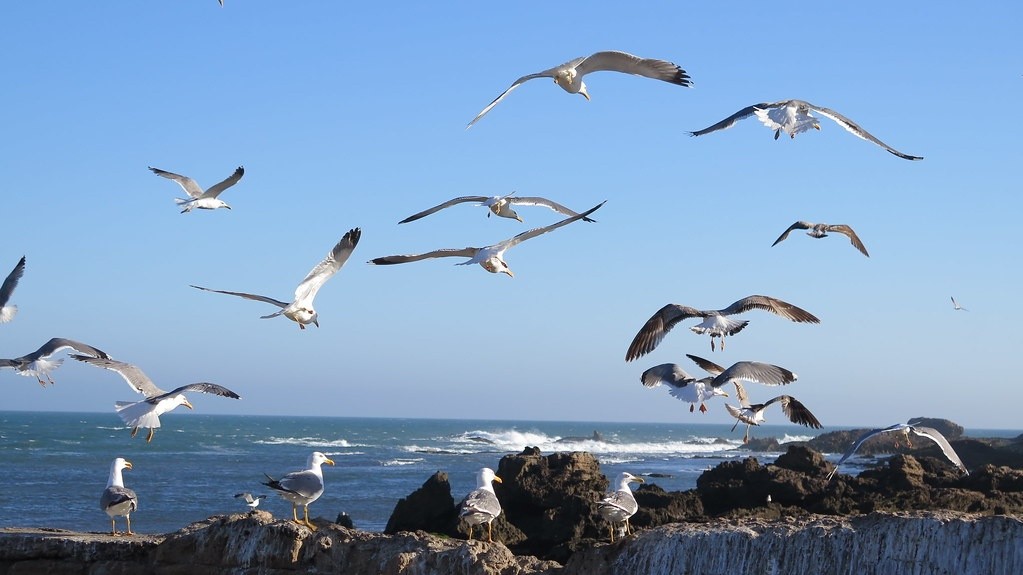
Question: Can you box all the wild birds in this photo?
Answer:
[950,295,971,314]
[624,296,822,363]
[146,164,245,214]
[641,354,824,445]
[592,472,645,546]
[366,191,608,279]
[683,98,925,162]
[457,467,502,543]
[826,423,969,482]
[188,226,362,330]
[464,51,694,131]
[1,255,243,538]
[232,451,335,531]
[771,220,872,260]
